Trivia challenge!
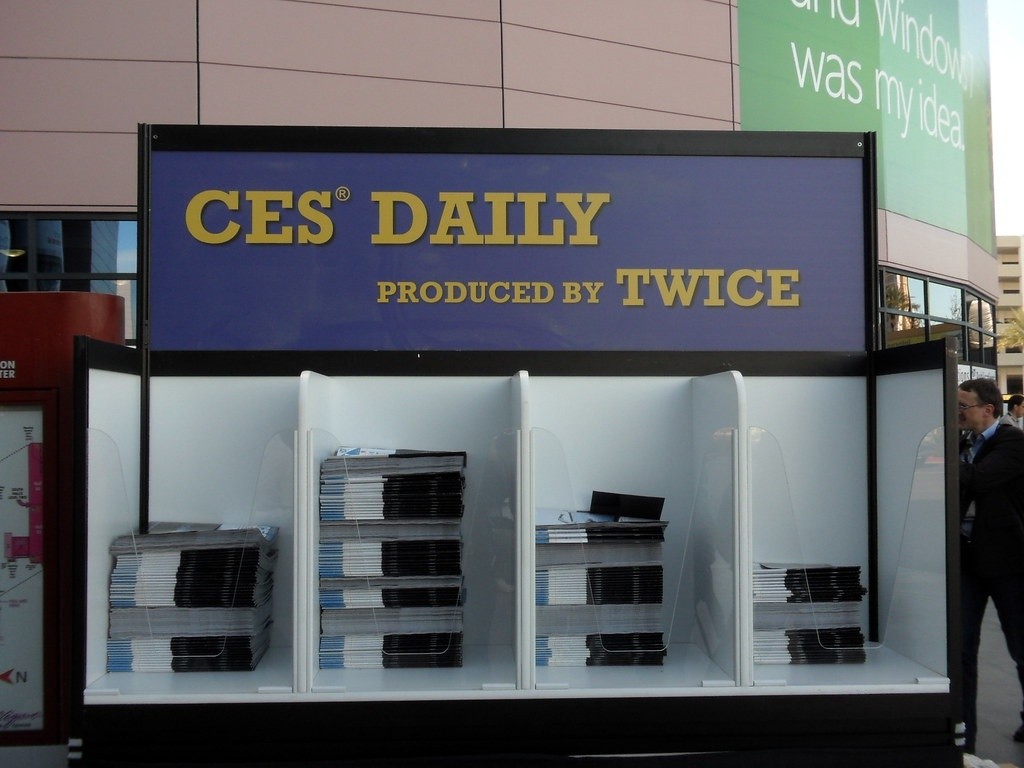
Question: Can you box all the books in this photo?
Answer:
[319,447,468,668]
[535,520,668,666]
[106,522,279,673]
[753,563,866,664]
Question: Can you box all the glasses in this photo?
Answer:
[958,402,988,412]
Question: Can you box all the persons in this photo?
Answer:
[999,394,1024,429]
[959,379,1024,754]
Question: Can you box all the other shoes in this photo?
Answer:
[1013,725,1024,742]
